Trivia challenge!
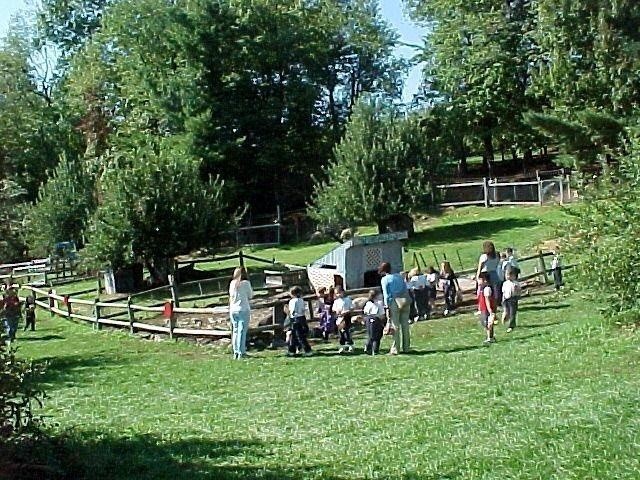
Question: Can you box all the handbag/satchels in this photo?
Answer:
[395,291,412,309]
[382,318,396,335]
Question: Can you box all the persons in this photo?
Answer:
[229,266,252,359]
[475,241,520,343]
[282,262,460,356]
[551,247,563,290]
[4,288,24,342]
[22,295,35,331]
